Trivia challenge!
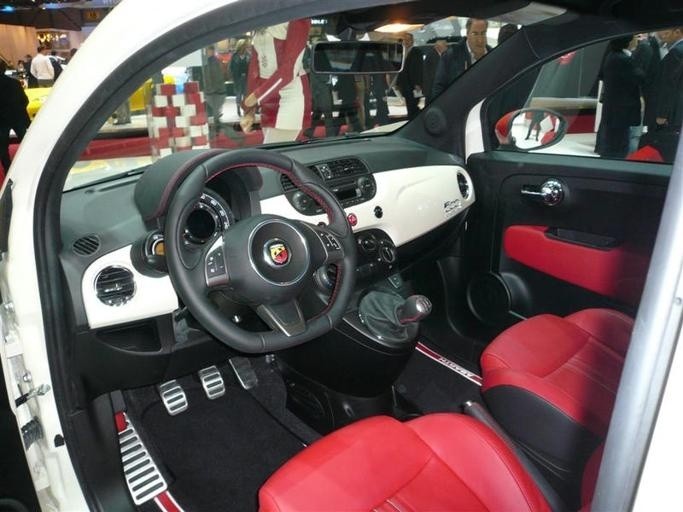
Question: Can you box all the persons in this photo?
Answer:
[229,37,254,119]
[302,15,519,142]
[202,44,243,147]
[589,24,681,163]
[17,46,132,127]
[0,54,33,179]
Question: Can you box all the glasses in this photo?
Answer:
[471,32,485,37]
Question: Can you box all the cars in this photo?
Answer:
[0,0,683,508]
[22,68,174,125]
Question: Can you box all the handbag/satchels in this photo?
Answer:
[638,128,680,162]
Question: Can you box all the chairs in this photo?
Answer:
[259,406,591,512]
[478,307,635,501]
